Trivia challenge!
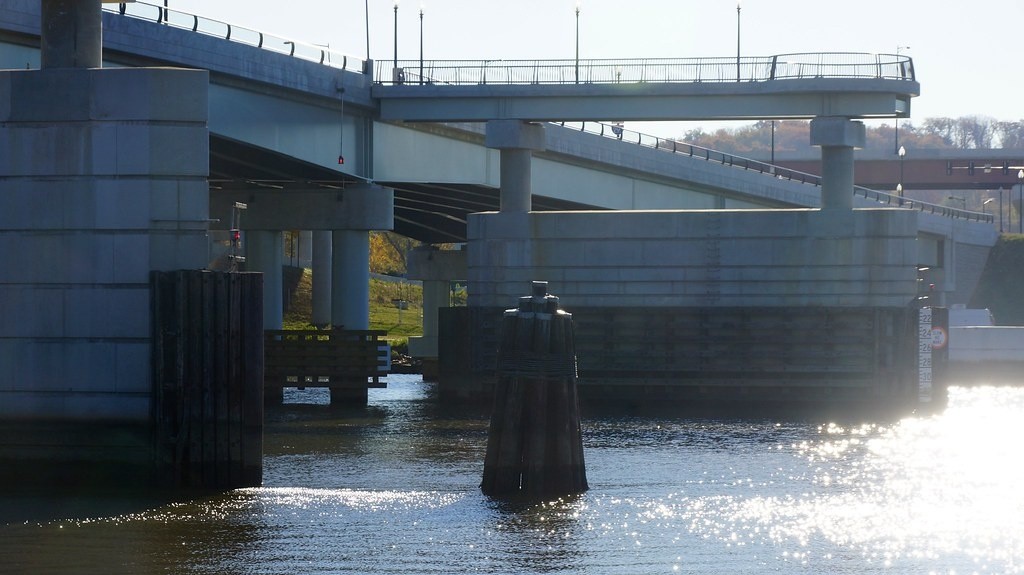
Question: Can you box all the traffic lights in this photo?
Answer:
[231,229,240,240]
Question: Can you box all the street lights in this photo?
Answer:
[1018,168,1024,235]
[897,144,906,209]
[416,4,426,84]
[572,2,583,83]
[947,196,967,211]
[392,0,401,66]
[981,196,995,223]
[735,0,742,82]
